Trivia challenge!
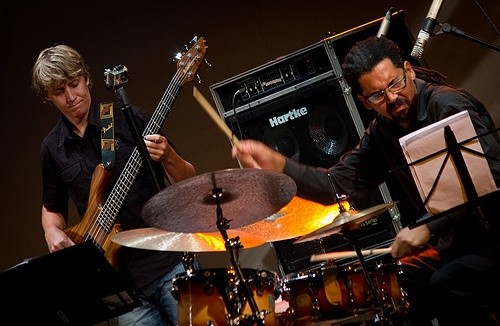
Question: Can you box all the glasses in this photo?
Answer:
[364,68,407,105]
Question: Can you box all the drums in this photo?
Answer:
[172,265,279,326]
[283,259,410,326]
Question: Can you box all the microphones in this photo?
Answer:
[410,0,444,60]
[377,7,395,37]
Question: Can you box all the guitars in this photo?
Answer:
[63,35,211,266]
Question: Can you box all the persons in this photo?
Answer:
[32,44,197,326]
[231,36,500,326]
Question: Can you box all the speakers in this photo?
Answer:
[209,10,436,276]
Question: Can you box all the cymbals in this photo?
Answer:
[109,167,352,253]
[291,198,401,246]
